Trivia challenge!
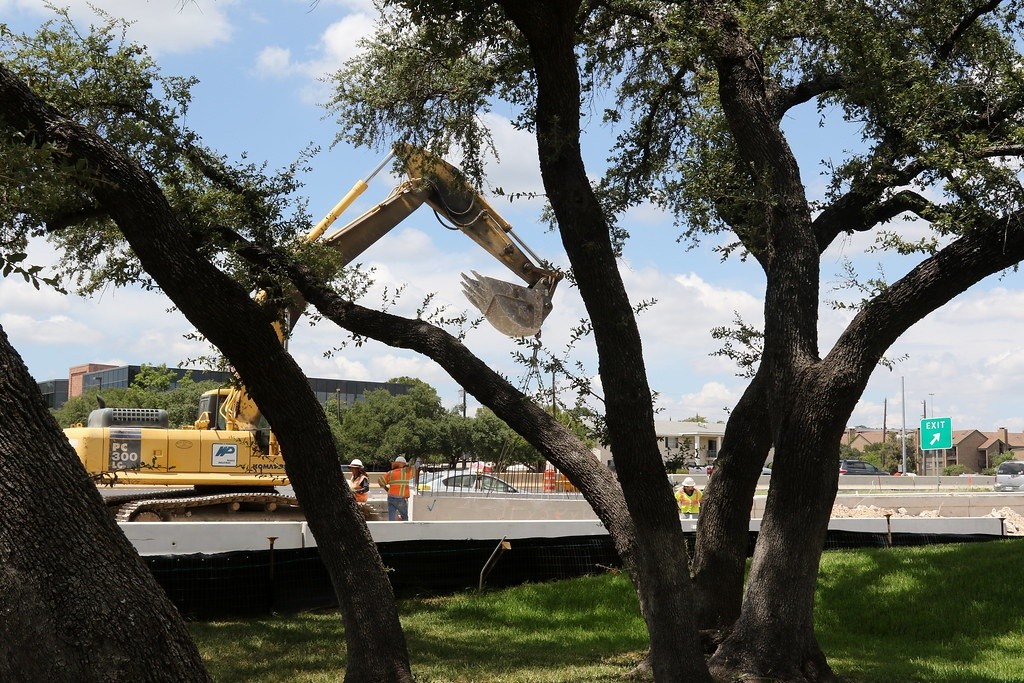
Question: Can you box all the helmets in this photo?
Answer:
[393,456,407,465]
[351,458,365,468]
[681,477,696,486]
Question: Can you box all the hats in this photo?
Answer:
[347,465,362,469]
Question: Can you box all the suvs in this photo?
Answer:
[995,461,1024,492]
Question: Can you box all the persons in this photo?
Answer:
[378,457,420,521]
[675,478,702,519]
[348,459,369,502]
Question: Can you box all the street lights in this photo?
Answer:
[336,387,342,421]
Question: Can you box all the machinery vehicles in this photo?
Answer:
[58,128,567,517]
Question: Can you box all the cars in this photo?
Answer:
[687,457,773,475]
[837,459,891,475]
[405,470,527,494]
